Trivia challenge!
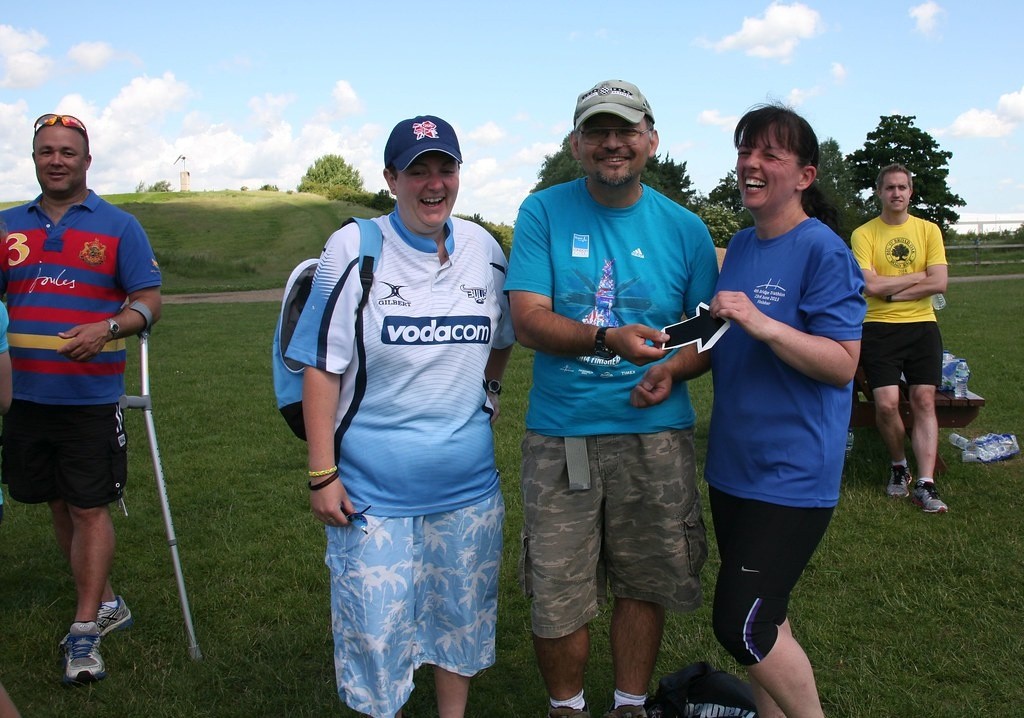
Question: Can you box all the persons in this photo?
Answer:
[0,114,162,718]
[698,105,865,718]
[502,80,721,718]
[849,163,947,513]
[284,114,516,718]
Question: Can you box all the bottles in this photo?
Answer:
[845,430,855,460]
[961,450,978,461]
[931,292,946,310]
[949,434,968,449]
[942,350,950,360]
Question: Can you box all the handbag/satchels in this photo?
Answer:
[644,662,758,718]
[273,216,384,441]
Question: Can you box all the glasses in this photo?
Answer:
[578,127,653,146]
[34,114,88,142]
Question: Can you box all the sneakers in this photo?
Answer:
[58,621,106,682]
[96,596,133,637]
[911,480,948,513]
[887,463,912,497]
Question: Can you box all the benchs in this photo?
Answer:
[899,380,986,407]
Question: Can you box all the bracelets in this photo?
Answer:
[886,294,893,302]
[306,467,339,490]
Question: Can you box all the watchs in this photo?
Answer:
[487,378,502,395]
[106,319,119,339]
[595,327,616,360]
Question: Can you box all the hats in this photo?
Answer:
[384,115,463,171]
[574,80,655,132]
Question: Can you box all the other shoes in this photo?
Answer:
[603,701,647,718]
[547,700,591,718]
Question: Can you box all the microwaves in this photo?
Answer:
[954,359,968,397]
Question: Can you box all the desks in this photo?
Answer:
[849,359,980,470]
